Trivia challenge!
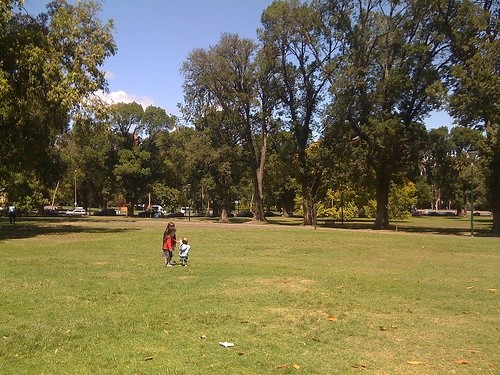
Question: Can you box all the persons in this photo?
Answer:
[178,238,191,267]
[163,222,177,264]
[8,203,16,224]
[162,227,179,267]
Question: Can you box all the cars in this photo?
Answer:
[93,208,116,216]
[151,204,166,215]
[193,212,205,216]
[139,211,154,217]
[168,211,184,217]
[237,209,253,216]
[265,211,276,216]
[66,208,86,217]
